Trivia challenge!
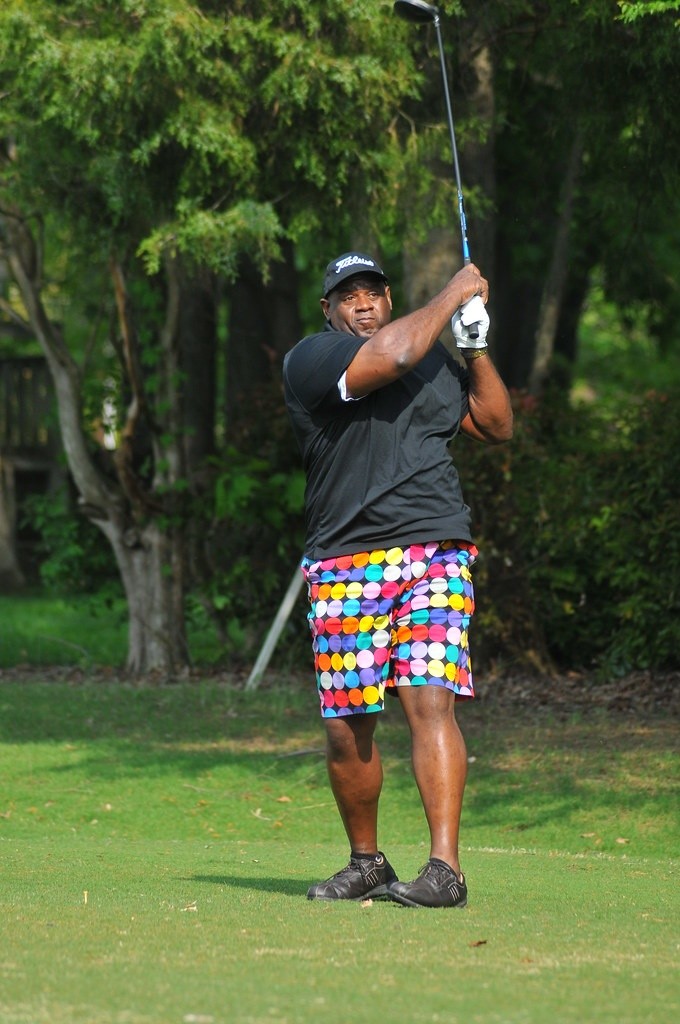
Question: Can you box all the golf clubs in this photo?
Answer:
[393,0,479,339]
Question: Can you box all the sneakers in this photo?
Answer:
[388,857,467,908]
[307,850,399,901]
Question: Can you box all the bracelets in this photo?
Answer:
[460,348,488,359]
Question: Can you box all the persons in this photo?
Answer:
[283,251,512,908]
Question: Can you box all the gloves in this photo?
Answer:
[451,294,490,349]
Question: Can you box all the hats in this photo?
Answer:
[322,253,389,298]
